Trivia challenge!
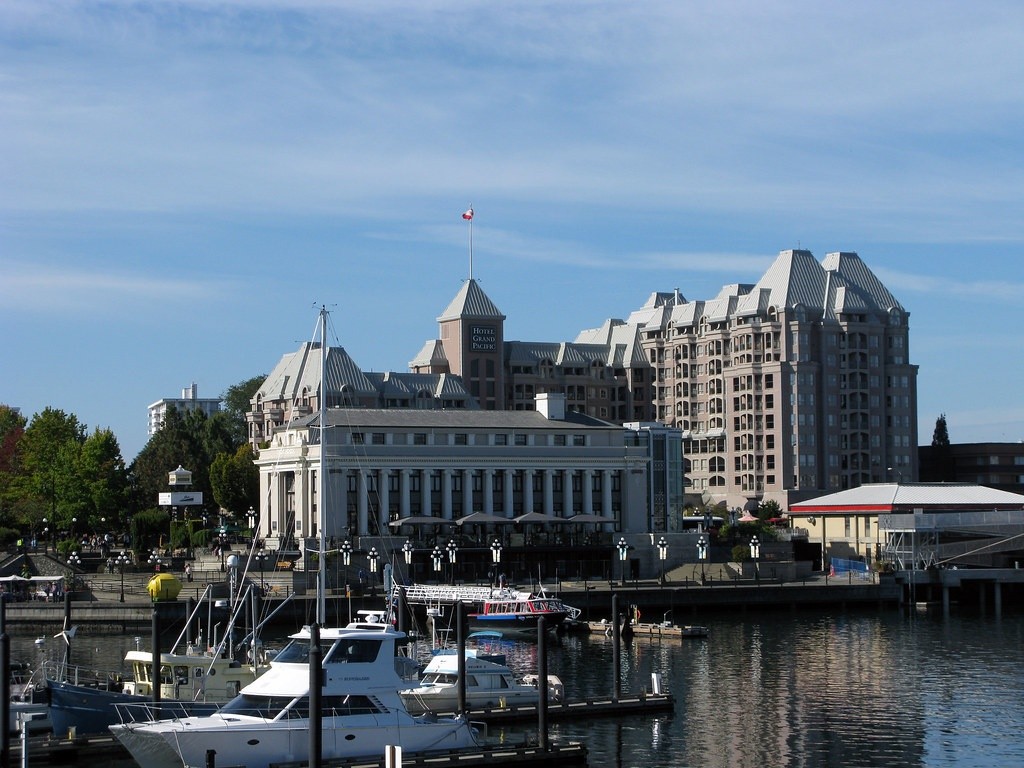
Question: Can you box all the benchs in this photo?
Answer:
[276,561,295,571]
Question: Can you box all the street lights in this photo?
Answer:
[219,526,228,573]
[616,537,632,585]
[446,539,459,586]
[255,548,269,597]
[367,546,381,597]
[66,551,82,600]
[431,546,444,585]
[489,539,504,586]
[696,535,709,586]
[656,537,669,587]
[113,550,131,604]
[402,540,415,584]
[147,551,161,576]
[340,540,354,594]
[749,534,762,583]
[245,505,258,548]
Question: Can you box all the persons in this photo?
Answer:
[160,637,263,685]
[184,564,193,583]
[16,533,232,561]
[44,584,50,597]
[51,582,57,603]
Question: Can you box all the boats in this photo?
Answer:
[105,610,483,767]
[397,652,566,710]
[0,527,278,741]
[467,599,571,643]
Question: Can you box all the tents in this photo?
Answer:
[386,512,619,547]
[0,574,34,602]
